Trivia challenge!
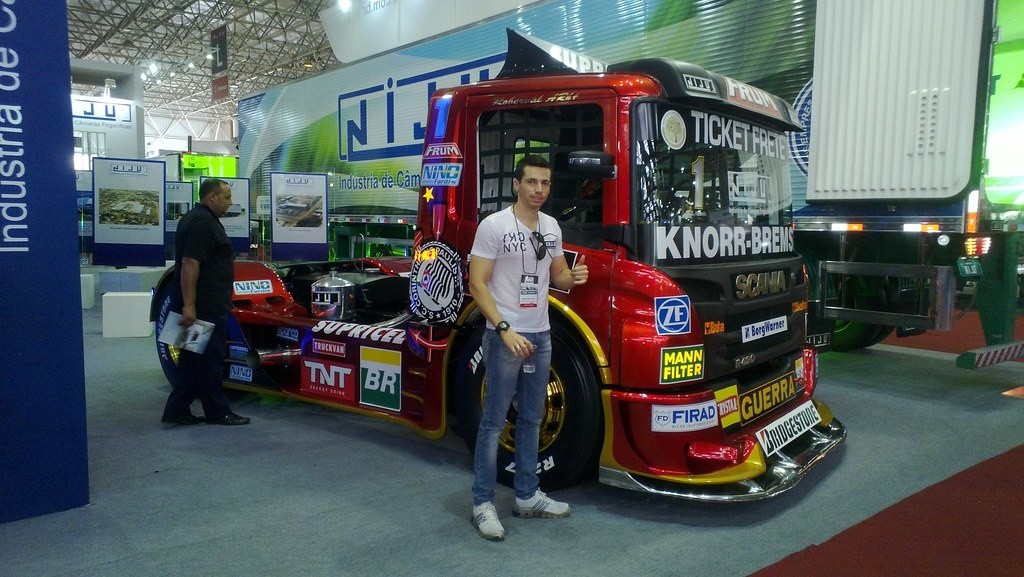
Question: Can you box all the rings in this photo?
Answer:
[516,345,520,349]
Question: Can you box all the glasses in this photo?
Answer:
[529,231,547,261]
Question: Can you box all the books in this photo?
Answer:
[158,311,215,354]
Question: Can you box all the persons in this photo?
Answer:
[160,178,251,426]
[468,155,588,541]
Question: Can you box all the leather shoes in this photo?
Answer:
[161,414,205,426]
[206,411,250,425]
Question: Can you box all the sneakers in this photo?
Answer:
[512,487,571,519]
[471,501,505,540]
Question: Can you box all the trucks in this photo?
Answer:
[149,28,847,503]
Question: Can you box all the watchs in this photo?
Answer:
[496,321,510,335]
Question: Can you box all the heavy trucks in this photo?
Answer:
[237,2,1024,369]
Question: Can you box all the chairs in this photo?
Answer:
[540,146,584,220]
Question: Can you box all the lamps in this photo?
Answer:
[155,51,214,84]
